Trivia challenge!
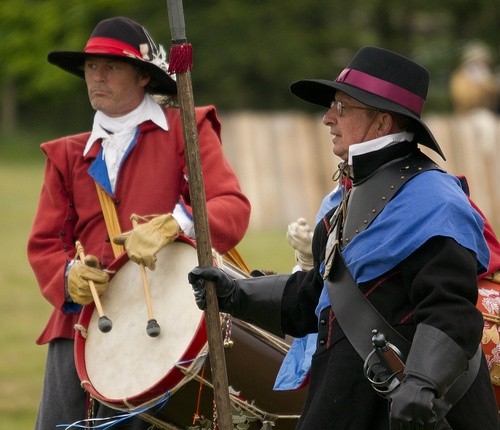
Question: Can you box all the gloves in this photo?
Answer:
[387,322,469,429]
[186,264,293,340]
[66,253,109,306]
[110,213,184,272]
[288,219,320,274]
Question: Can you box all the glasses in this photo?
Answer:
[320,95,369,116]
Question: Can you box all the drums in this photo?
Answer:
[72,234,306,430]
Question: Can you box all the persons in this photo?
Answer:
[27,17,251,430]
[187,45,500,430]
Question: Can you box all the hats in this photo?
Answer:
[47,15,178,98]
[288,45,448,163]
